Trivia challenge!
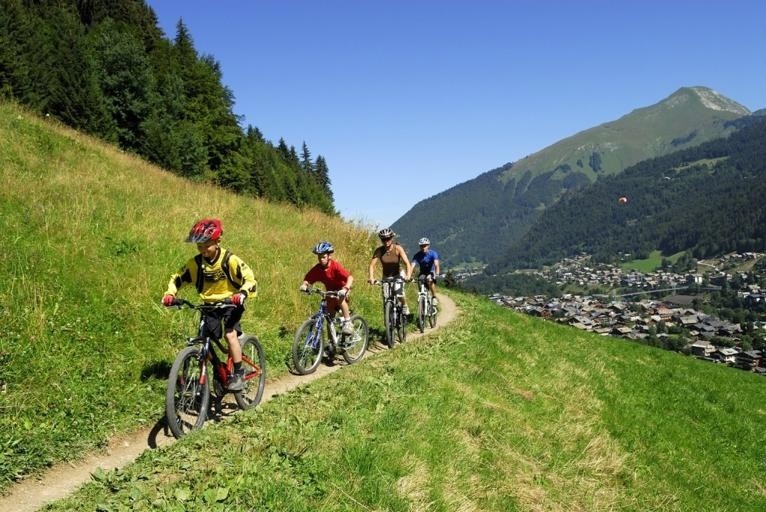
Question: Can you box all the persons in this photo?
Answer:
[161,219,257,392]
[370,229,412,316]
[410,237,440,316]
[300,241,355,358]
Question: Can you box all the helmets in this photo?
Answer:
[314,242,333,254]
[190,218,222,243]
[419,237,430,245]
[379,229,394,239]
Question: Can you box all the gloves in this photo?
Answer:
[164,295,173,306]
[232,293,245,305]
[338,290,346,296]
[300,284,307,291]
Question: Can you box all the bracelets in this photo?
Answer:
[343,286,349,290]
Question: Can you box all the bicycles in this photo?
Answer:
[162,296,266,442]
[410,273,447,333]
[366,277,418,350]
[291,286,370,375]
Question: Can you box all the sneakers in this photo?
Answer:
[402,305,410,315]
[433,298,438,305]
[228,374,247,390]
[342,322,353,334]
